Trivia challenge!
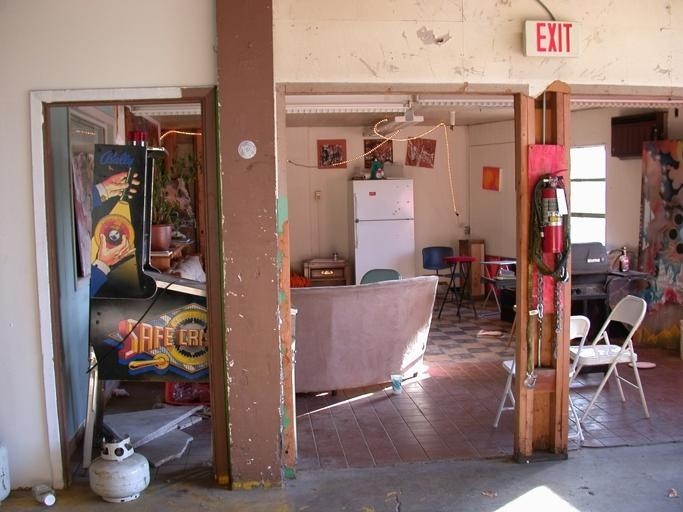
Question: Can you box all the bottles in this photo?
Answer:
[33,485,58,509]
[618,245,631,273]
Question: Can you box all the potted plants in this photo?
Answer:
[151,176,181,250]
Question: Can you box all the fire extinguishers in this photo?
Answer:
[534,169,569,254]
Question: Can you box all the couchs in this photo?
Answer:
[290,276,440,394]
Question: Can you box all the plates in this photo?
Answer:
[628,361,657,369]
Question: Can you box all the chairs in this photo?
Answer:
[361,269,400,285]
[420,247,456,313]
[492,294,651,445]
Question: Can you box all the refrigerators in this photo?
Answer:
[349,178,417,284]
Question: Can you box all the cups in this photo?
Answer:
[391,375,402,394]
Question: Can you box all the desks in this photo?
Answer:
[605,268,649,290]
[437,256,478,321]
[478,261,517,315]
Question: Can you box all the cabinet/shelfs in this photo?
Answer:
[151,240,191,272]
[459,239,485,298]
[610,111,669,160]
[303,259,353,287]
